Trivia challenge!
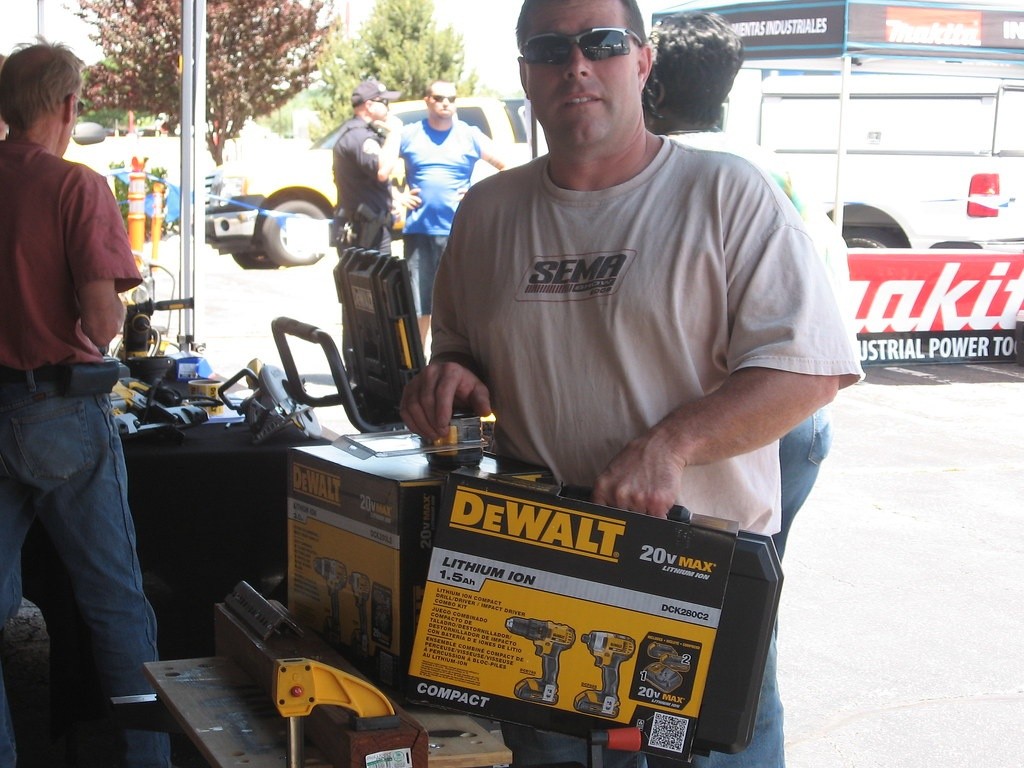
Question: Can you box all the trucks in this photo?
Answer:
[666,71,1024,250]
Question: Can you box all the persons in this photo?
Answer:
[328,79,402,258]
[644,8,850,768]
[0,54,9,140]
[401,0,866,768]
[0,39,172,767]
[394,80,510,353]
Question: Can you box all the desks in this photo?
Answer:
[144,657,512,768]
[22,370,331,701]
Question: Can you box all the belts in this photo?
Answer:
[0,365,64,381]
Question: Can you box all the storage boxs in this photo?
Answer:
[333,247,426,422]
[399,461,784,760]
[286,436,553,703]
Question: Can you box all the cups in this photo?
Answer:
[187,380,225,416]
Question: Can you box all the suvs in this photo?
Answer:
[190,91,551,271]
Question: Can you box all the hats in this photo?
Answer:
[352,80,402,106]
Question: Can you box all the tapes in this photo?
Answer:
[186,379,224,399]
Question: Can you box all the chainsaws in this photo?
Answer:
[218,358,324,444]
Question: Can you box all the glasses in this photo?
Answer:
[372,98,389,107]
[430,95,456,103]
[522,27,642,64]
